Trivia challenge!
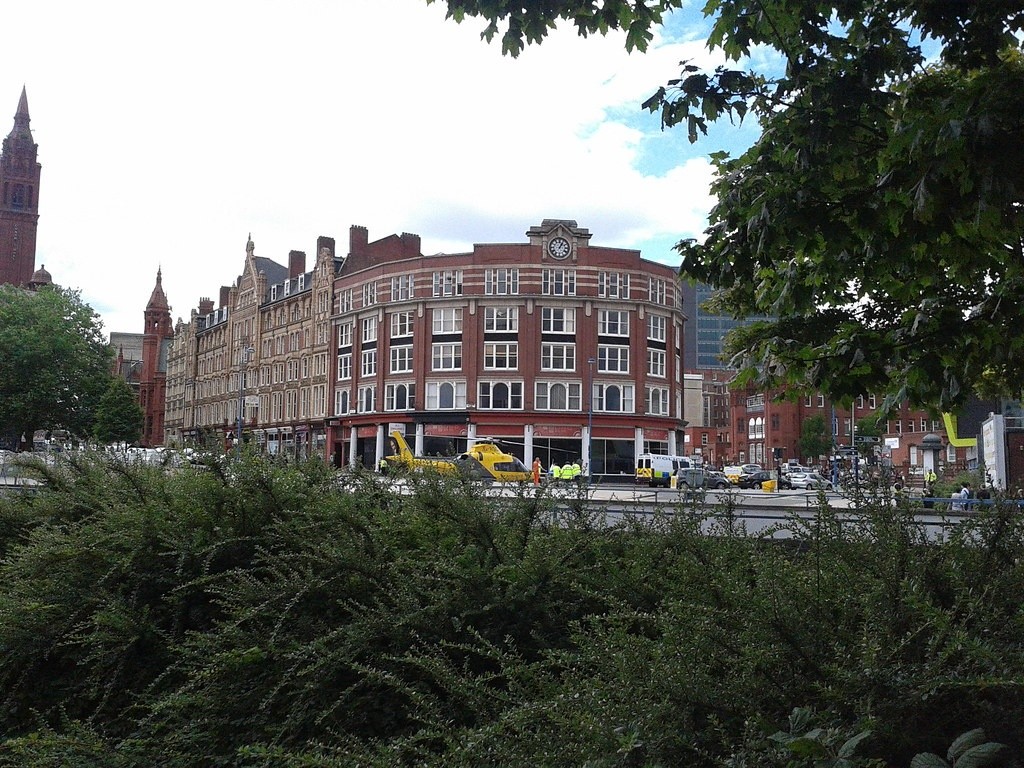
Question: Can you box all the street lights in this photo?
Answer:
[129,360,145,382]
[237,347,255,452]
[587,358,597,485]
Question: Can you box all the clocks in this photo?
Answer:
[547,235,572,260]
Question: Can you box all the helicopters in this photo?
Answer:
[385,427,580,490]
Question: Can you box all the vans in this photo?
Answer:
[790,466,812,472]
[635,452,693,487]
[743,464,763,475]
[723,467,744,484]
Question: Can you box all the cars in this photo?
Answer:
[738,471,791,491]
[792,473,834,490]
[66,443,221,464]
[671,467,730,490]
[709,471,728,484]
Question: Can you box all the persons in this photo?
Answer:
[534,457,547,488]
[976,483,991,511]
[553,461,583,488]
[924,468,937,487]
[946,481,974,511]
